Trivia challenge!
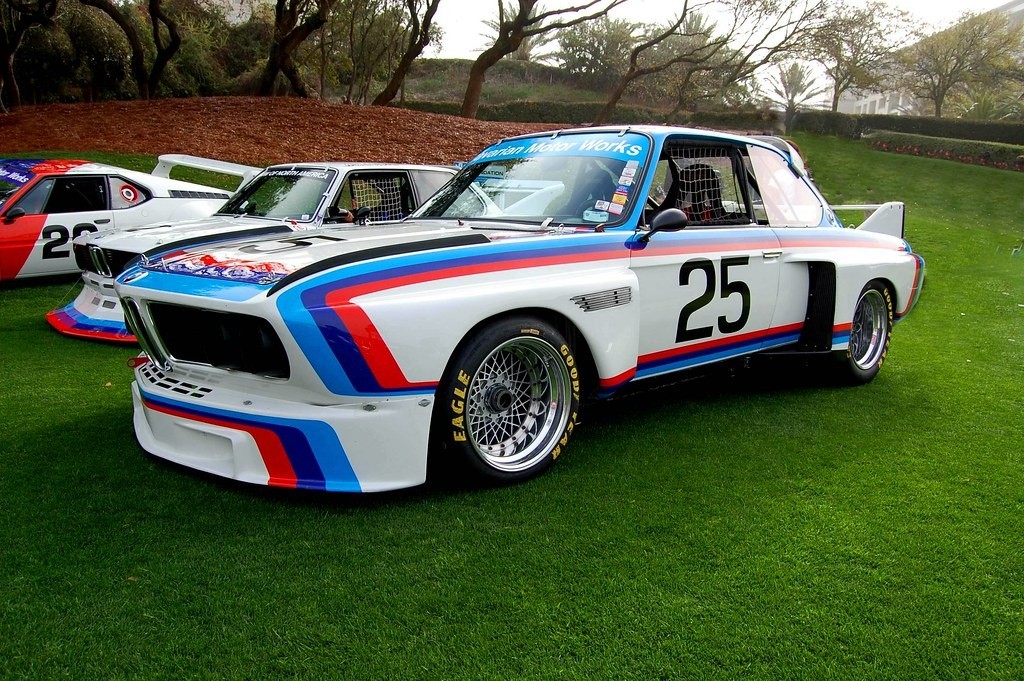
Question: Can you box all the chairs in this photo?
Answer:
[674,163,728,225]
[566,168,615,217]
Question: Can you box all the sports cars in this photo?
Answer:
[0,152,268,280]
[42,159,565,342]
[111,123,924,497]
